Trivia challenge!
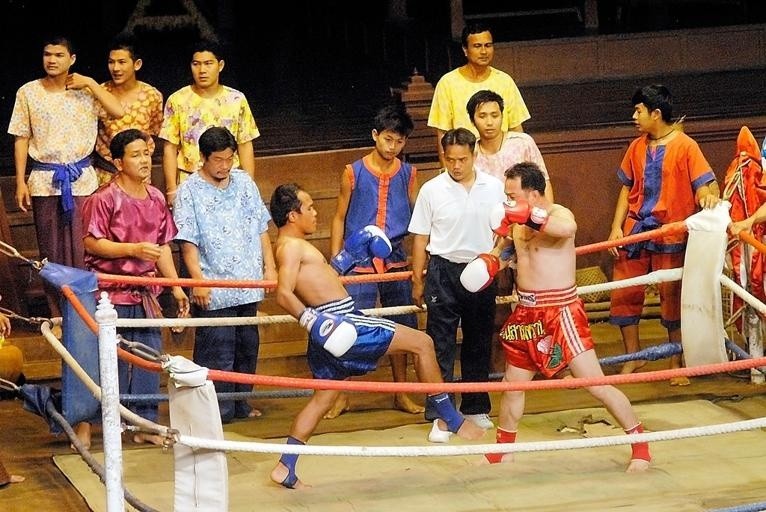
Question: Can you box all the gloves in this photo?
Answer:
[487,199,550,239]
[329,225,392,276]
[458,252,501,294]
[297,306,359,359]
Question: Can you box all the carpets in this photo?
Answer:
[50,392,766,512]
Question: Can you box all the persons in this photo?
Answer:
[68,129,191,455]
[427,21,531,175]
[407,127,506,445]
[466,88,557,206]
[269,182,486,493]
[158,39,262,335]
[0,312,24,399]
[90,37,164,189]
[7,33,125,338]
[458,160,652,476]
[322,104,427,420]
[173,126,279,422]
[607,83,722,387]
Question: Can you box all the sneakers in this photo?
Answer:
[457,408,495,430]
[428,418,453,443]
